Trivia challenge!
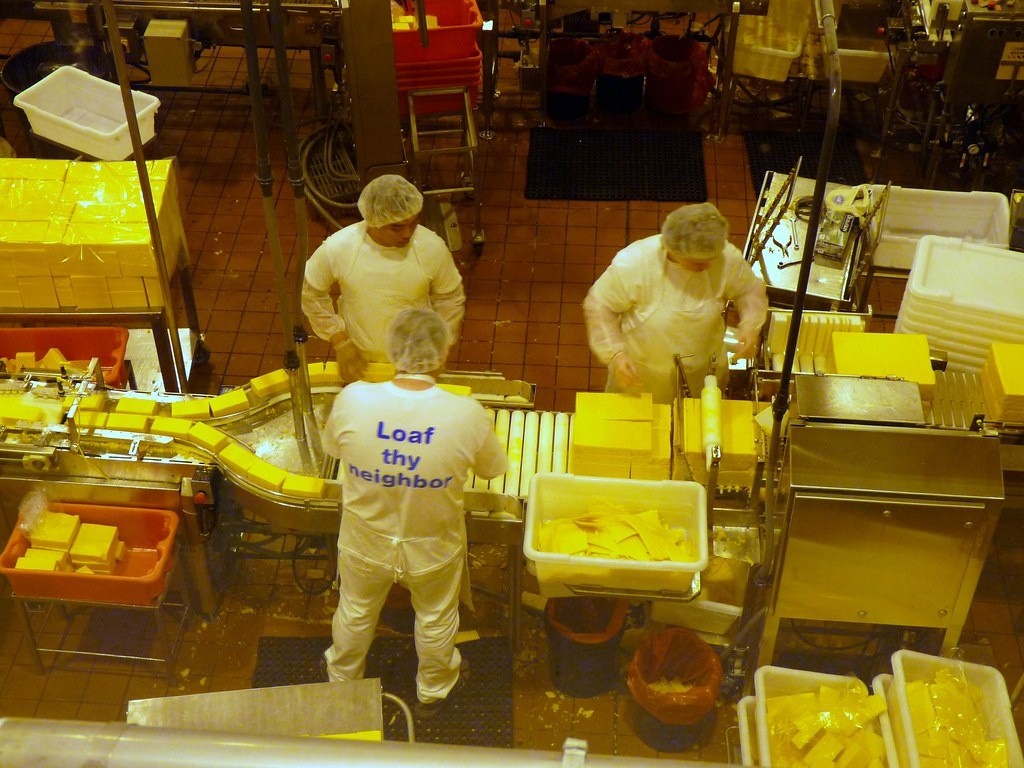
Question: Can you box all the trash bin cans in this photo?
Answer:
[543,597,627,700]
[629,628,723,752]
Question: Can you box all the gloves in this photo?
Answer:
[612,351,635,388]
[725,329,756,360]
[334,340,367,381]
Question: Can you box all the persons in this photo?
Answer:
[301,174,467,383]
[325,308,509,710]
[582,202,770,405]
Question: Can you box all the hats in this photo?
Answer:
[357,175,423,227]
[661,202,728,257]
[387,309,454,373]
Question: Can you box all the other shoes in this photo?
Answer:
[415,660,471,718]
[320,647,329,682]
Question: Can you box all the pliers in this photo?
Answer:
[772,235,792,257]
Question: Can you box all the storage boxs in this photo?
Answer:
[732,0,812,83]
[0,503,179,605]
[394,42,483,71]
[12,64,161,160]
[648,557,750,635]
[398,76,482,116]
[524,473,709,595]
[737,695,761,768]
[867,183,1010,271]
[871,673,900,768]
[886,649,1024,768]
[893,234,1024,374]
[819,35,895,84]
[396,61,482,79]
[397,71,482,87]
[392,0,484,64]
[755,665,869,768]
